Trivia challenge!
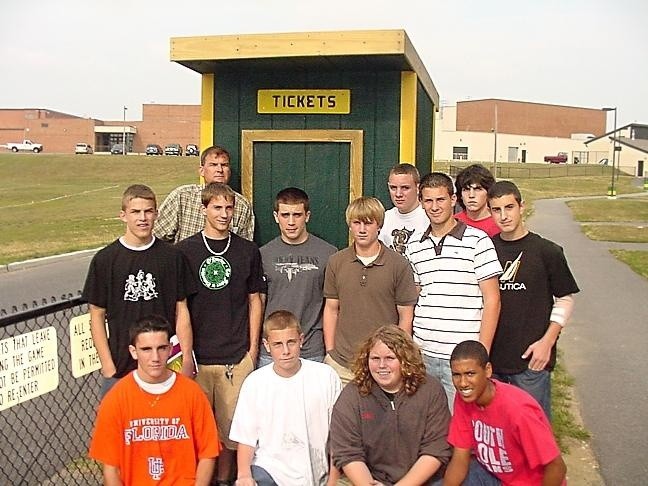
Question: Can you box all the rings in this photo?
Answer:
[192,371,196,376]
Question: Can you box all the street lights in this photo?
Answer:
[602,107,618,197]
[123,105,128,155]
[490,127,496,180]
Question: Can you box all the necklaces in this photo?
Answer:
[201,230,232,255]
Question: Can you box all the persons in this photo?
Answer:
[329,325,453,486]
[376,163,433,256]
[153,146,257,244]
[319,196,416,388]
[175,183,266,486]
[226,309,343,486]
[255,185,339,370]
[89,313,223,486]
[487,181,582,423]
[449,162,503,239]
[80,184,199,396]
[402,171,500,419]
[439,339,568,486]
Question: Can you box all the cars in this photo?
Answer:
[111,144,128,155]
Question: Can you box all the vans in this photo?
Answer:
[74,143,93,154]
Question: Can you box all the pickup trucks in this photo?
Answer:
[7,139,43,153]
[544,151,579,165]
[164,144,183,156]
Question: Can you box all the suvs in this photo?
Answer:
[145,144,163,156]
[184,143,199,157]
